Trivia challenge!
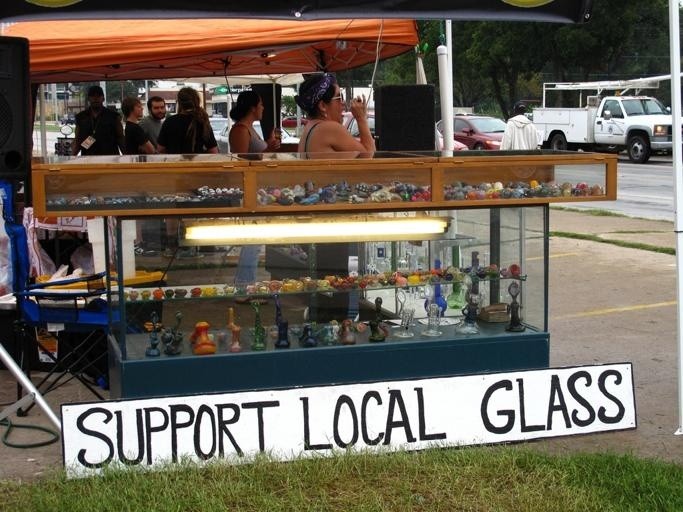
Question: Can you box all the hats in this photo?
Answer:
[88,86,103,95]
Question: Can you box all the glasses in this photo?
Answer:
[329,91,344,103]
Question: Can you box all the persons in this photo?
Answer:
[292,72,378,324]
[498,101,544,151]
[226,89,282,306]
[120,95,162,257]
[68,85,127,155]
[138,94,167,155]
[155,87,219,260]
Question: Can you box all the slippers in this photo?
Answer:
[234,297,268,305]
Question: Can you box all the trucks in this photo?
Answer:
[530,94,682,165]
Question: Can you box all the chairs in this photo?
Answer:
[0,184,123,417]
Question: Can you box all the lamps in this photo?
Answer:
[175,214,455,247]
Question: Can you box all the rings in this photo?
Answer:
[357,99,361,102]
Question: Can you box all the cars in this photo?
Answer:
[208,111,508,151]
[60,112,76,126]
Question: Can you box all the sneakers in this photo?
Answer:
[134,240,203,260]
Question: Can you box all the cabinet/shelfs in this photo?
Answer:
[30,149,618,400]
